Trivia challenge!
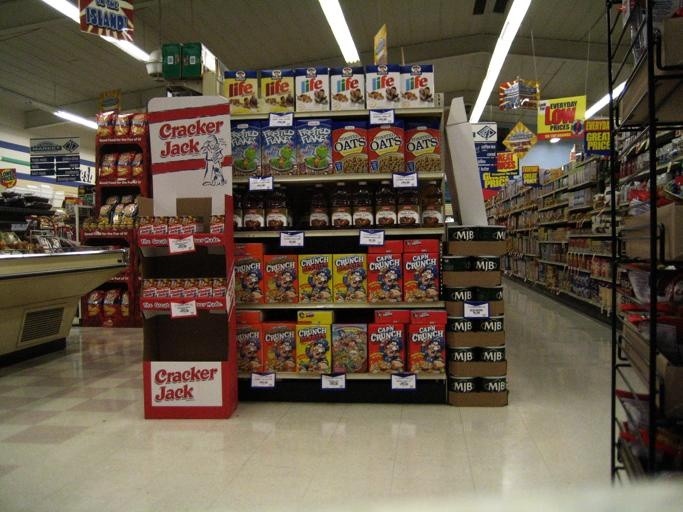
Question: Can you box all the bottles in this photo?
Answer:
[421,180,443,226]
[308,184,329,229]
[232,184,242,229]
[266,183,288,228]
[398,187,420,226]
[354,184,373,227]
[243,188,264,229]
[331,183,352,229]
[375,180,397,226]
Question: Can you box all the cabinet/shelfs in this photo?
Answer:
[78,134,149,328]
[603,1,683,510]
[234,95,448,393]
[137,194,236,421]
[488,159,614,328]
